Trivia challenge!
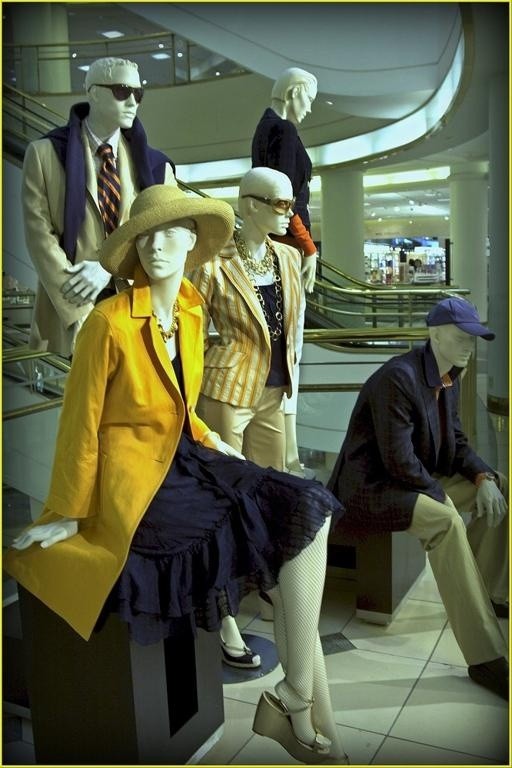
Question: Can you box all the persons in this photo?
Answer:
[250,67,323,474]
[2,182,362,765]
[24,55,177,358]
[369,269,383,283]
[190,167,307,668]
[323,292,510,703]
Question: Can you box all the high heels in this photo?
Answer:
[252,677,330,764]
[219,631,260,669]
[258,590,273,621]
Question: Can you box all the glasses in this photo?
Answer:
[88,83,144,103]
[242,194,297,215]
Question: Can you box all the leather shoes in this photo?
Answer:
[469,656,509,702]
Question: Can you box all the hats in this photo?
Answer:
[425,297,495,341]
[99,184,236,278]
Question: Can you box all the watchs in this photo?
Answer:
[475,472,502,489]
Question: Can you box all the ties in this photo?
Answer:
[96,144,120,240]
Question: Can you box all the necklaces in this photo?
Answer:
[233,232,287,342]
[152,301,180,345]
